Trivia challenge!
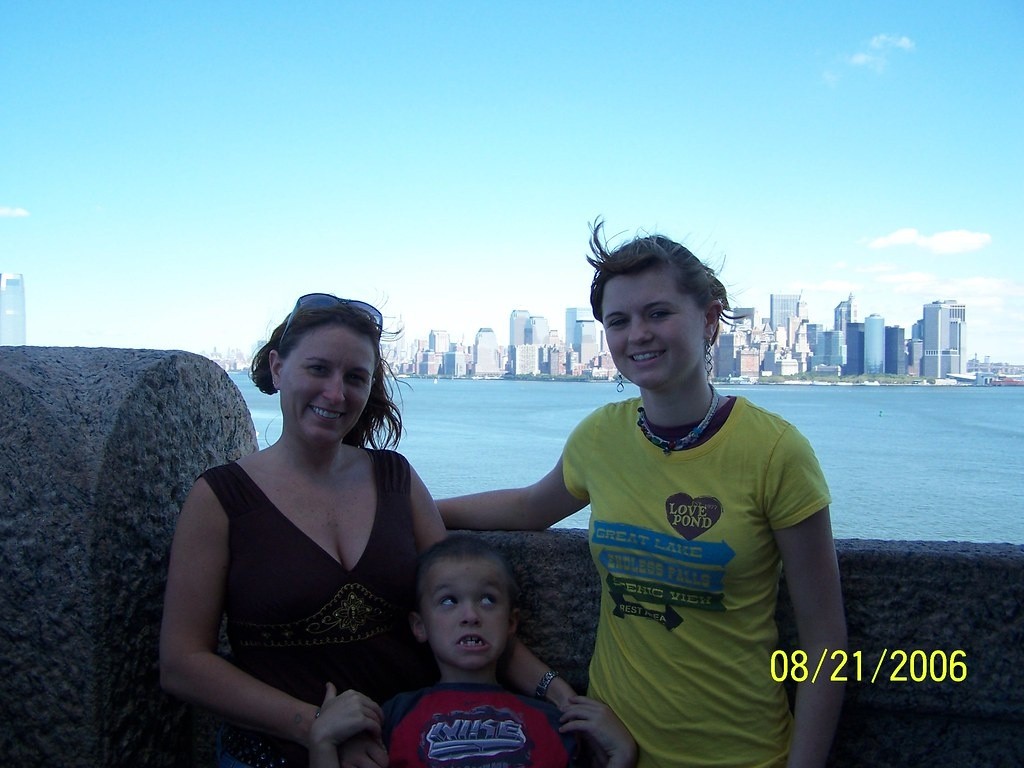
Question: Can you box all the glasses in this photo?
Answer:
[279,293,383,343]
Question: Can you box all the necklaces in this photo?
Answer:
[637,383,720,454]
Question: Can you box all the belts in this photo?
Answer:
[223,727,288,768]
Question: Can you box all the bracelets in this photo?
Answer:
[536,670,558,698]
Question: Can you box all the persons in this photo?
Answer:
[434,235,848,768]
[307,528,638,768]
[159,294,578,768]
